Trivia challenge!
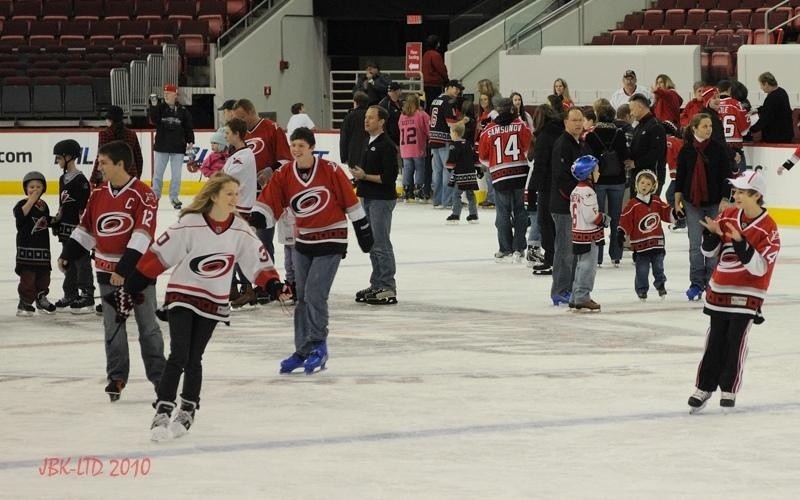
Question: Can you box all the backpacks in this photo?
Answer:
[590,130,622,177]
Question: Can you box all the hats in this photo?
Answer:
[217,99,236,109]
[664,120,680,132]
[701,85,720,107]
[623,70,636,77]
[725,169,766,196]
[448,79,465,91]
[104,286,145,325]
[210,128,229,151]
[164,85,176,92]
[387,83,399,92]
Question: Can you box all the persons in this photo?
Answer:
[11,171,54,314]
[57,137,169,393]
[248,125,374,371]
[46,81,317,318]
[340,32,800,313]
[123,173,293,441]
[687,170,780,415]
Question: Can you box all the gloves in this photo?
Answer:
[360,238,375,253]
[601,214,611,227]
[448,168,455,174]
[476,168,483,179]
[615,233,627,249]
[527,194,538,209]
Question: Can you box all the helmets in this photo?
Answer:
[23,172,46,195]
[54,139,80,155]
[187,159,201,173]
[106,106,124,121]
[571,154,599,182]
[635,169,659,195]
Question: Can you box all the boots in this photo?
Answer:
[403,183,433,199]
[175,394,200,431]
[668,218,685,229]
[527,245,551,270]
[149,400,177,429]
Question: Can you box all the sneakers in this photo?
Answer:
[479,200,495,207]
[659,286,667,295]
[467,214,478,220]
[494,249,525,257]
[357,286,396,299]
[229,278,277,308]
[569,299,600,309]
[720,391,736,407]
[280,341,328,369]
[17,286,103,313]
[435,198,466,220]
[687,284,703,298]
[638,293,648,298]
[688,388,712,406]
[172,197,182,208]
[105,381,125,395]
[552,291,571,302]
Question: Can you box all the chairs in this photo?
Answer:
[0,0,272,129]
[586,0,800,143]
